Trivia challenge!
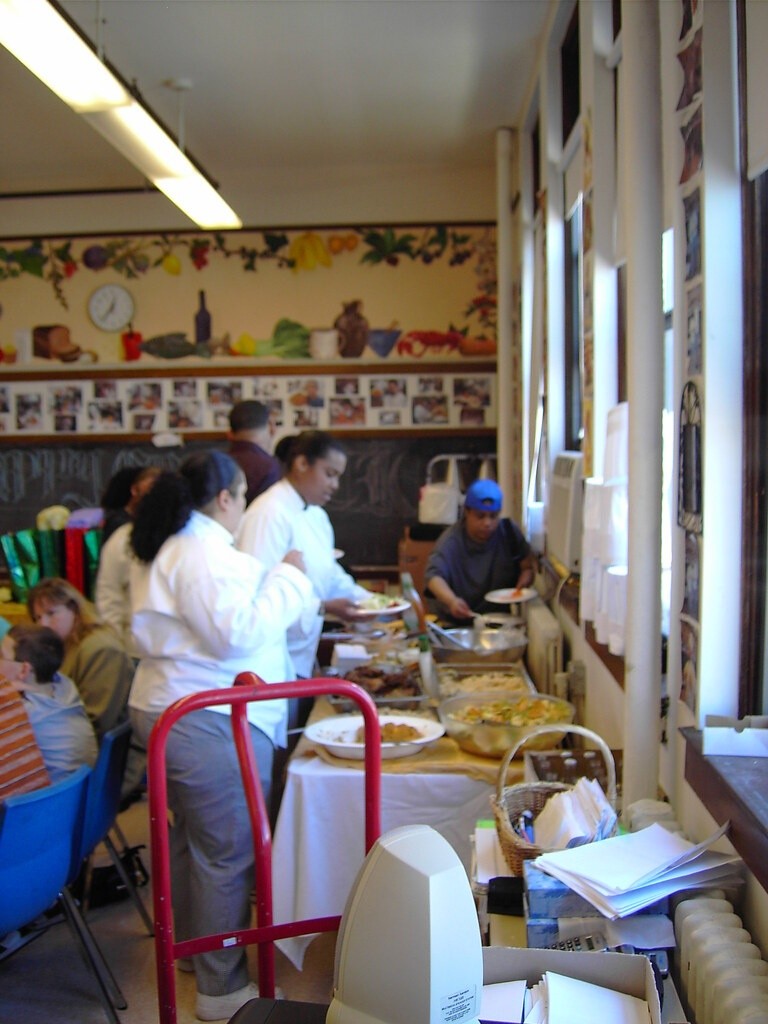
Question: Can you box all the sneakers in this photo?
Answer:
[179,958,197,971]
[194,981,281,1021]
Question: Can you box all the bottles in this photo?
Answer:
[417,635,443,708]
[398,572,429,651]
[533,749,624,787]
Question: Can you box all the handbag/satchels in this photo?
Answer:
[88,844,149,911]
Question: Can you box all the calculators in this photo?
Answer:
[636,950,669,979]
[540,930,607,952]
[603,944,636,954]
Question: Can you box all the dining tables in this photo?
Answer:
[265,599,591,971]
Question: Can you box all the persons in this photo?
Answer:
[230,430,374,906]
[217,398,284,507]
[0,620,100,807]
[423,479,538,623]
[125,444,313,1021]
[383,380,405,405]
[304,379,323,407]
[95,451,179,658]
[25,577,137,735]
[414,398,447,422]
[336,399,356,418]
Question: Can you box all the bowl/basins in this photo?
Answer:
[437,690,577,758]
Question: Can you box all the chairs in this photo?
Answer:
[1,718,161,1024]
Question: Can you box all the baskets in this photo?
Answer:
[491,723,616,878]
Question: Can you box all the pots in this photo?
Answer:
[430,617,527,670]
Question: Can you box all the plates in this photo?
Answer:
[303,713,446,760]
[484,588,537,603]
[346,600,411,616]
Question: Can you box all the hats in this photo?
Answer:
[465,480,504,511]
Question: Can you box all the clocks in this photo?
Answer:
[89,285,135,332]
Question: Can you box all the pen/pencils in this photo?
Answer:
[514,810,540,841]
